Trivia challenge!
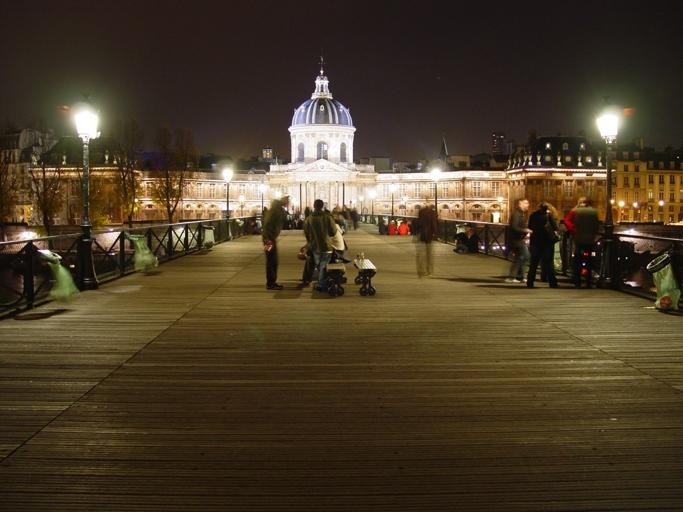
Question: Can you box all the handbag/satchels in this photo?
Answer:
[545,223,559,244]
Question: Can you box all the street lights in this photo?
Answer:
[431,169,442,215]
[617,200,663,221]
[496,196,505,223]
[596,114,616,290]
[239,196,246,217]
[359,197,364,216]
[72,111,101,290]
[388,183,396,215]
[369,190,377,214]
[259,184,269,212]
[222,168,236,240]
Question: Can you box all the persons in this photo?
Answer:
[261,197,289,289]
[304,199,337,286]
[413,204,441,279]
[304,207,310,219]
[453,223,478,254]
[261,207,268,229]
[282,206,300,230]
[376,219,416,235]
[503,196,599,289]
[324,205,360,231]
[297,210,345,260]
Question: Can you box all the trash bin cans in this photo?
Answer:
[646,253,681,311]
[29,250,80,305]
[201,226,215,249]
[554,235,563,272]
[126,234,159,273]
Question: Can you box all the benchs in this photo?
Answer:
[325,262,347,297]
[353,257,376,296]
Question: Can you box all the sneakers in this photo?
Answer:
[266,283,282,290]
[506,278,527,282]
